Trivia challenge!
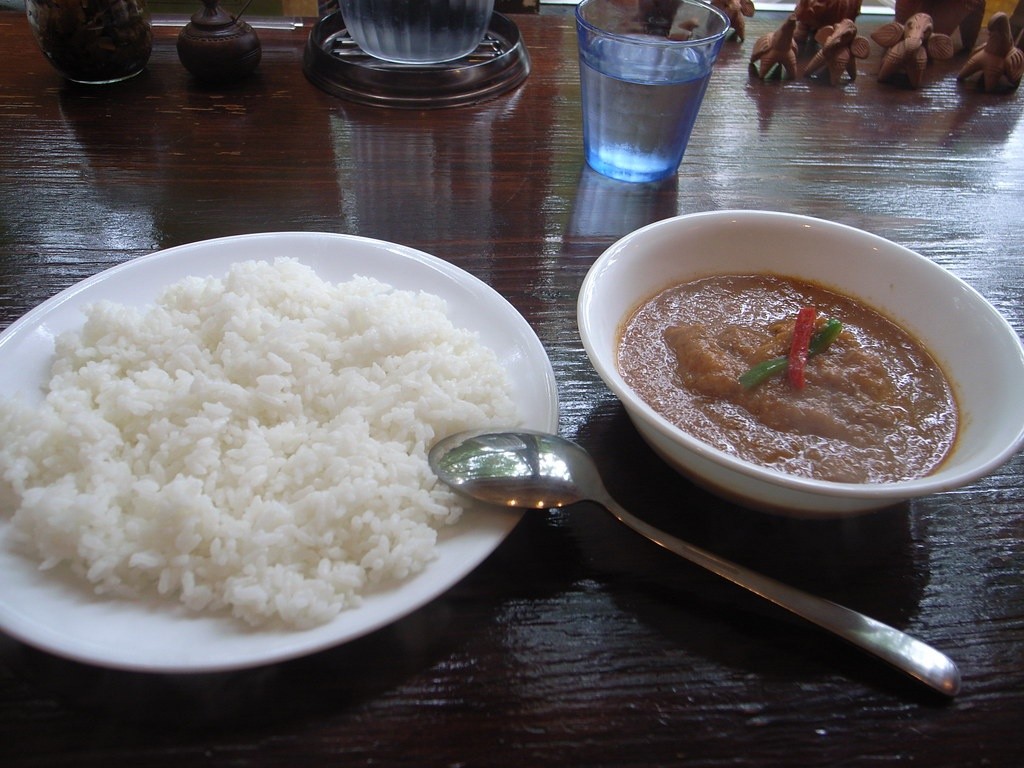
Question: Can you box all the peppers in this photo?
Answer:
[739,306,843,389]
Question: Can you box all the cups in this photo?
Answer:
[25,0,153,84]
[338,0,494,64]
[575,0,731,182]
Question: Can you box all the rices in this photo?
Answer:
[0,256,527,630]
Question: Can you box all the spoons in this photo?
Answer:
[429,429,962,699]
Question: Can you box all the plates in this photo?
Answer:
[0,232,559,673]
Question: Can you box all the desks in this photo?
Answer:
[0,0,1024,768]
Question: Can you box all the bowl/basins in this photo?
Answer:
[577,209,1023,522]
